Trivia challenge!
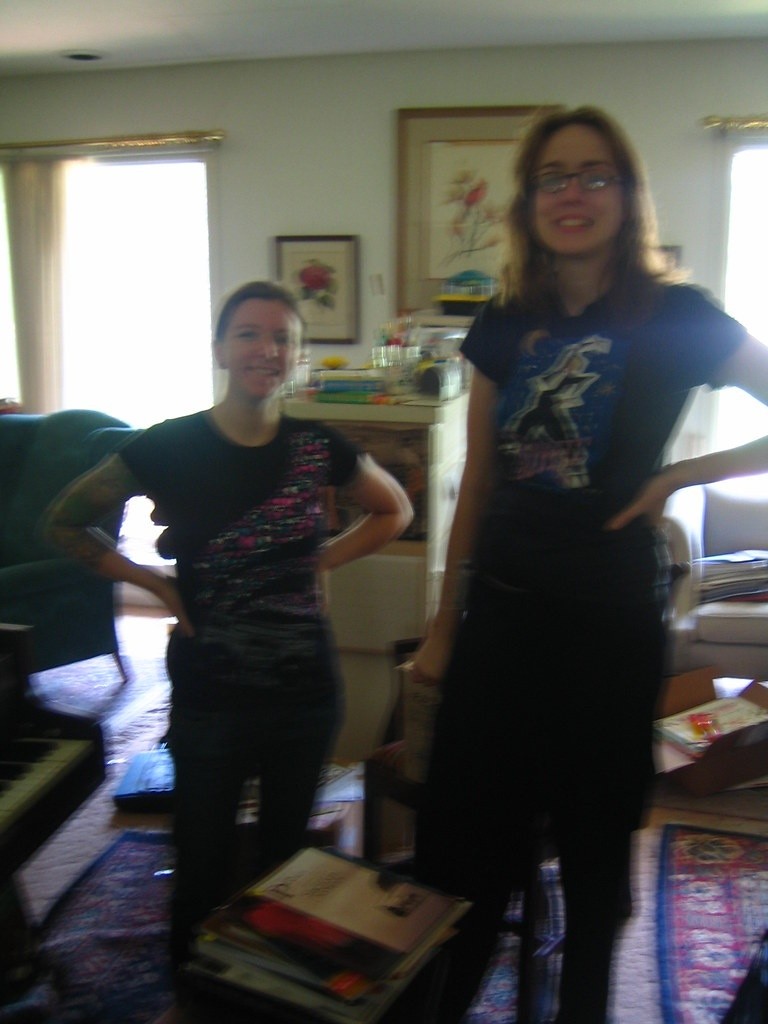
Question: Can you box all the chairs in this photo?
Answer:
[365,640,543,933]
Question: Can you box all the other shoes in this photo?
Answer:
[150,995,205,1024]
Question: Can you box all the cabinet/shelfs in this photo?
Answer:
[277,423,434,766]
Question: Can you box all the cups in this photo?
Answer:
[281,381,293,399]
[291,359,310,387]
[371,345,403,368]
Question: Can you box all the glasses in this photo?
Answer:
[533,169,626,193]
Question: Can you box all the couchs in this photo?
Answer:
[666,485,768,677]
[0,412,141,694]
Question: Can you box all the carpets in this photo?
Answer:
[0,830,566,1024]
[609,807,768,1024]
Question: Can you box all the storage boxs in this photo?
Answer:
[655,670,768,795]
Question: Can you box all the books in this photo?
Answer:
[310,368,416,404]
[192,842,474,1023]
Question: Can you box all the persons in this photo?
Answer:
[41,281,415,973]
[410,107,768,1024]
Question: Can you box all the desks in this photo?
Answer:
[150,846,456,1024]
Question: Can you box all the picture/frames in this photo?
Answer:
[273,237,360,344]
[396,105,561,315]
